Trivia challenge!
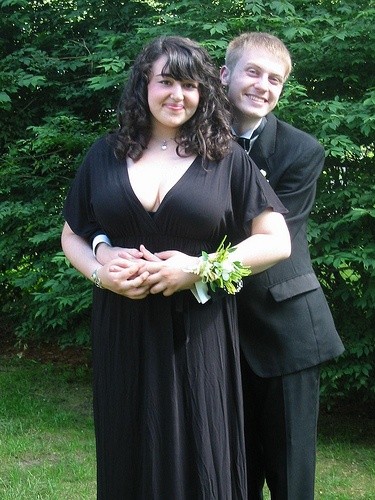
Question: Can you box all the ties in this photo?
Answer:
[237,138,250,154]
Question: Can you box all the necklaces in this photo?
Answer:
[154,132,177,149]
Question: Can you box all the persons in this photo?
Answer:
[62,37,291,500]
[92,33,346,500]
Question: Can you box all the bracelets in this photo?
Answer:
[92,268,103,288]
